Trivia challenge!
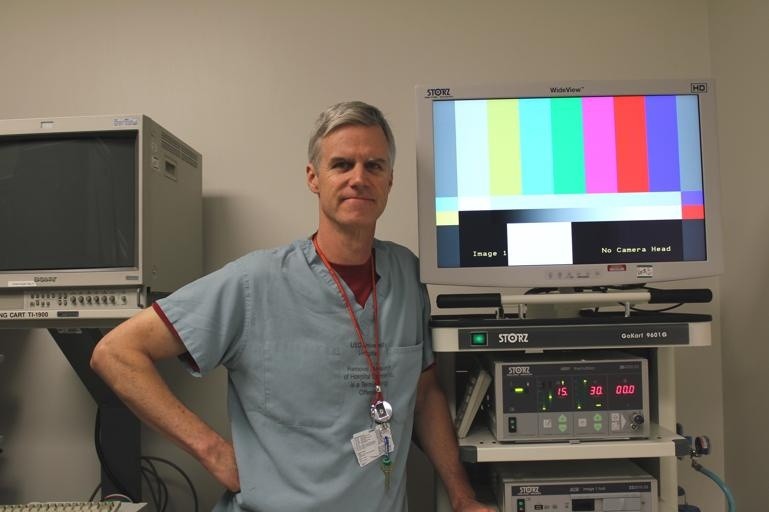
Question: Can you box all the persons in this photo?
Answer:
[90,101,494,512]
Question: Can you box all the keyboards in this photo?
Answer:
[0,501,120,512]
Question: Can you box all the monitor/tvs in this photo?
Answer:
[414,77,726,288]
[0,113,204,319]
